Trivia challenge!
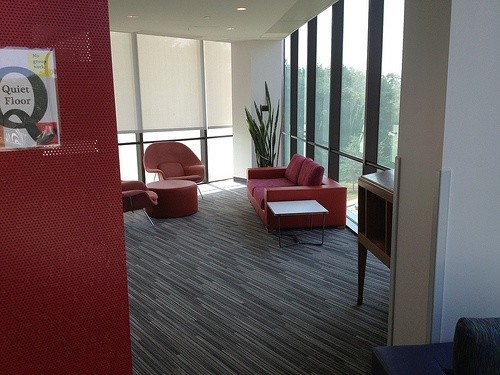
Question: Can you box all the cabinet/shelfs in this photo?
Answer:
[358,169,395,305]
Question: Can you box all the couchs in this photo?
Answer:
[373,317,500,375]
[247,153,346,230]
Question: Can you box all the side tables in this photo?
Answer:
[267,200,329,248]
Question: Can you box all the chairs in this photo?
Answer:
[120,180,158,230]
[143,141,206,200]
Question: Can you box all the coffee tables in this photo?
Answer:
[145,180,198,219]
